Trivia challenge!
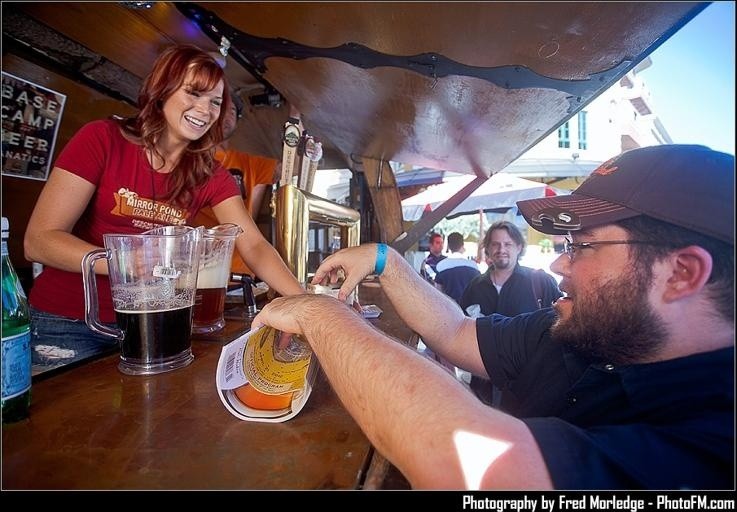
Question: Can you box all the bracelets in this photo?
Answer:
[374,242,388,276]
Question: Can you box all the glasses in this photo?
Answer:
[562,234,660,265]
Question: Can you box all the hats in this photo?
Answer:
[515,144,734,245]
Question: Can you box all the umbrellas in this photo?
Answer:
[403,174,579,253]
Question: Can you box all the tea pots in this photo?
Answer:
[81,221,245,377]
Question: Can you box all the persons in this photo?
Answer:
[461,219,562,408]
[183,89,321,292]
[419,235,488,289]
[249,144,733,490]
[23,42,309,376]
[433,231,479,308]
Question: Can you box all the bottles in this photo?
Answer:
[2,217,37,422]
